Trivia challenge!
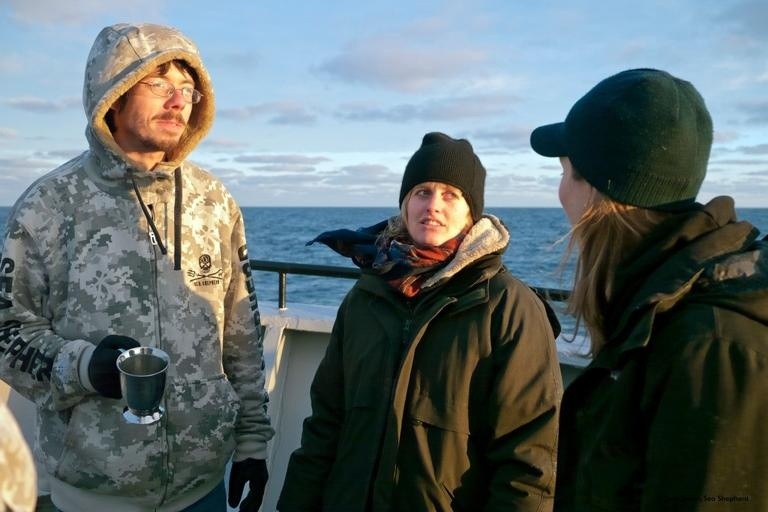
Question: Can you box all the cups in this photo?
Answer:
[116,346,170,426]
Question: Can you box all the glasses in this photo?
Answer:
[138,78,204,105]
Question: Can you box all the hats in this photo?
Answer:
[530,68,715,212]
[397,130,487,222]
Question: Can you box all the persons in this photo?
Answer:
[522,66,768,512]
[2,18,279,510]
[269,129,568,510]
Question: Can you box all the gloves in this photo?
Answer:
[89,334,141,401]
[228,458,269,512]
